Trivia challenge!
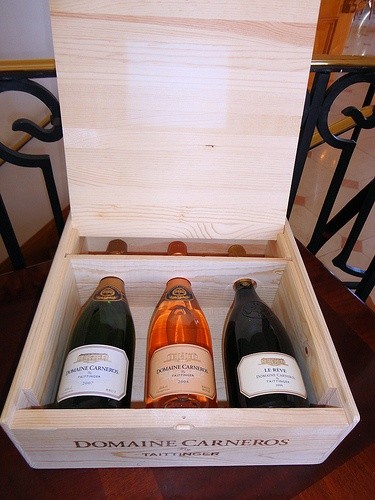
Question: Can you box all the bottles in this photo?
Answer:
[53,238,136,411]
[221,243,310,409]
[144,242,219,409]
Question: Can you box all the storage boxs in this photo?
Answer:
[0,0,359,467]
